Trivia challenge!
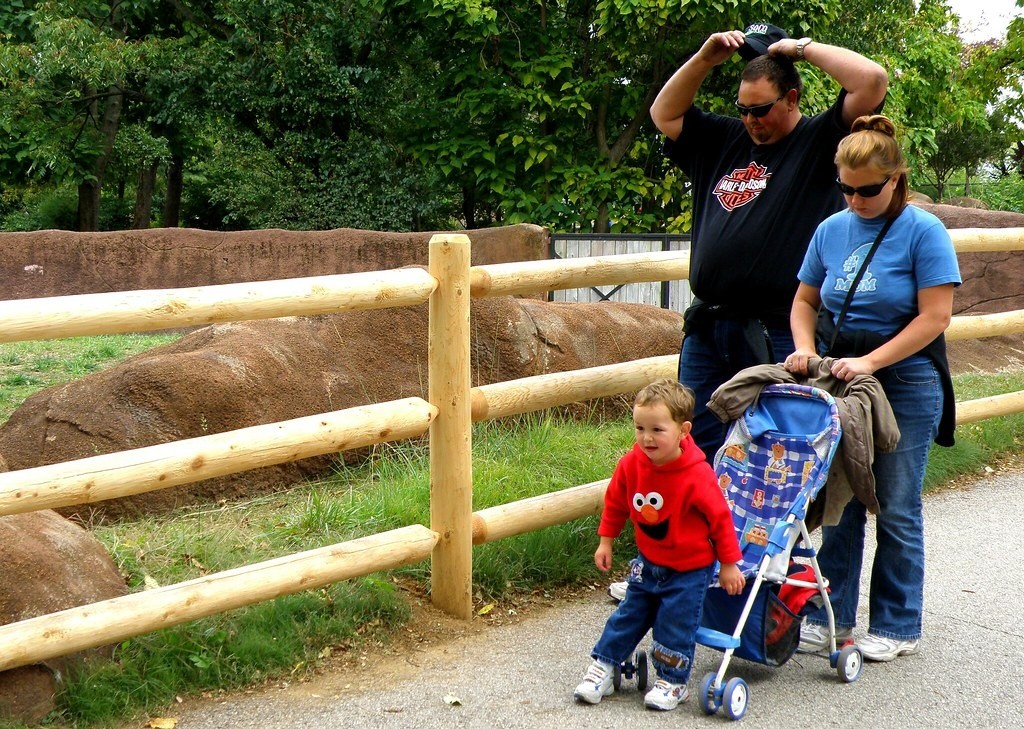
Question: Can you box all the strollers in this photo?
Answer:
[611,357,865,723]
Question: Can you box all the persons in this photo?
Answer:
[572,380,745,712]
[607,21,888,601]
[782,113,963,663]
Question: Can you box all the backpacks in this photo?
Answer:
[766,558,831,654]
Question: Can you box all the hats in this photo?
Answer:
[737,23,789,60]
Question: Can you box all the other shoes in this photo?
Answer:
[607,582,629,601]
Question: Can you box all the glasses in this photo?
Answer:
[735,94,785,117]
[836,176,891,197]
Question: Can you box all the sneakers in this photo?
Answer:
[574,661,615,704]
[796,622,853,654]
[855,632,920,662]
[644,678,690,711]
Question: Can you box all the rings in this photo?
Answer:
[788,362,792,366]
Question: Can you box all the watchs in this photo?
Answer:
[796,37,811,60]
